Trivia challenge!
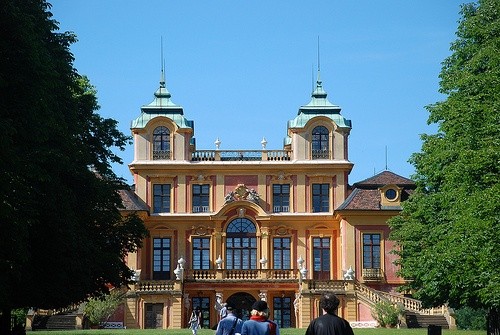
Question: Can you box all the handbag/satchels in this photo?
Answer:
[192,310,197,322]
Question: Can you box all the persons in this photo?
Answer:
[216,306,244,335]
[304,293,354,335]
[241,301,280,335]
[220,302,228,320]
[188,306,202,335]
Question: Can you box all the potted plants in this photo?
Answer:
[370,297,405,328]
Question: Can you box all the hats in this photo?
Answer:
[226,304,237,310]
[252,300,267,310]
